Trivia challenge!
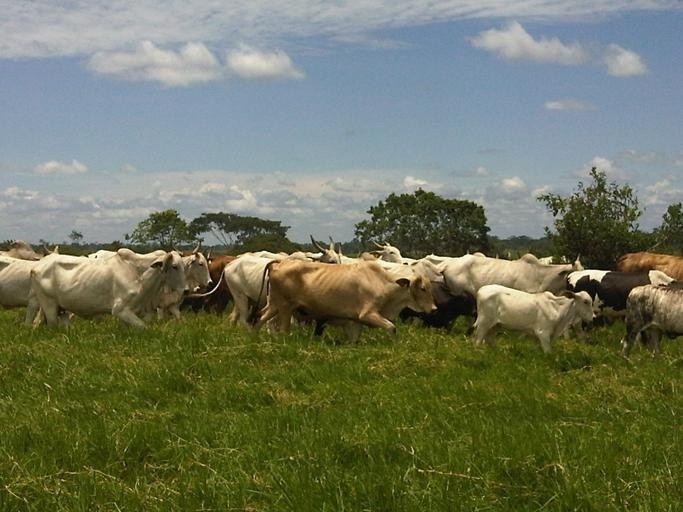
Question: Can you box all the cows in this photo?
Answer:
[1,234,682,360]
